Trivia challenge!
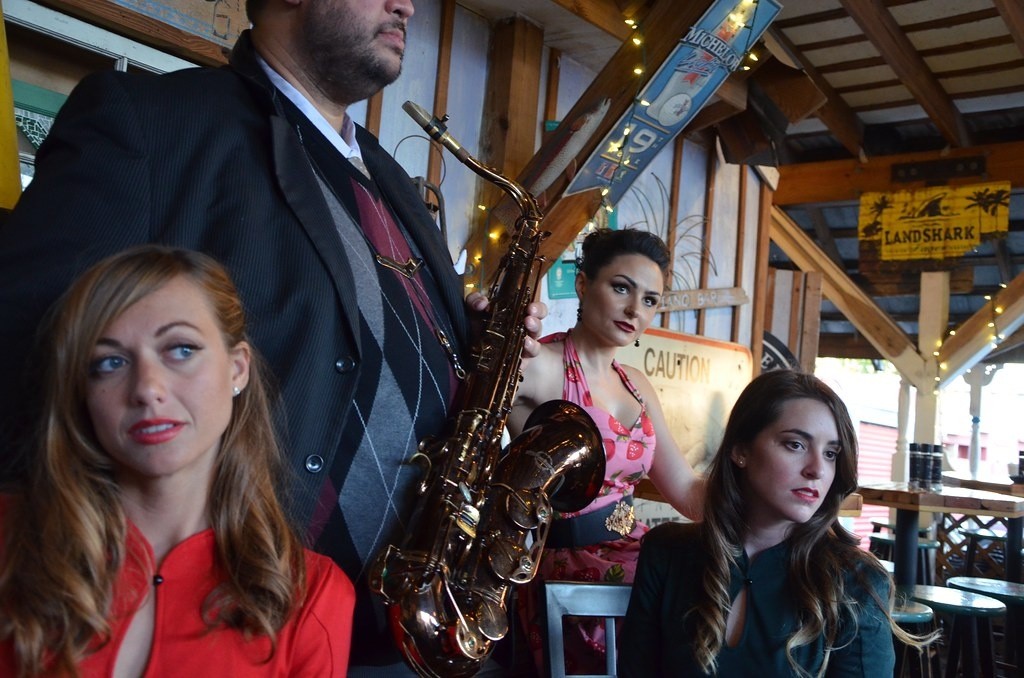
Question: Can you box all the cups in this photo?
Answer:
[909,443,920,488]
[919,443,932,491]
[932,445,943,491]
[1018,451,1024,478]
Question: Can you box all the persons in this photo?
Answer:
[0,0,548,678]
[501,226,711,678]
[0,246,357,678]
[615,368,943,678]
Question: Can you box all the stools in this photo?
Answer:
[869,516,1024,678]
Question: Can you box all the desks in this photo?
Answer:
[837,470,1024,596]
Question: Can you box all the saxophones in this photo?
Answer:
[369,99,608,677]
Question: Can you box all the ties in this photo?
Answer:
[345,150,372,182]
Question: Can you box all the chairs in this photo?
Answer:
[542,582,635,678]
